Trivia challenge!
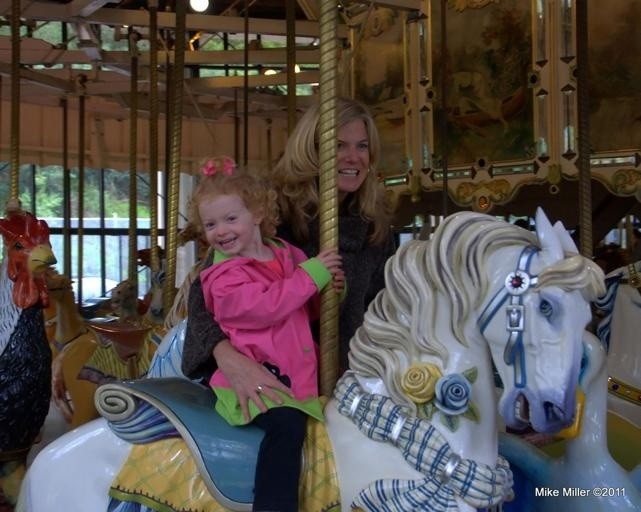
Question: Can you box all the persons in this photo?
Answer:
[181,97,395,424]
[189,155,346,507]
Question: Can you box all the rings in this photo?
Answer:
[256,386,265,393]
